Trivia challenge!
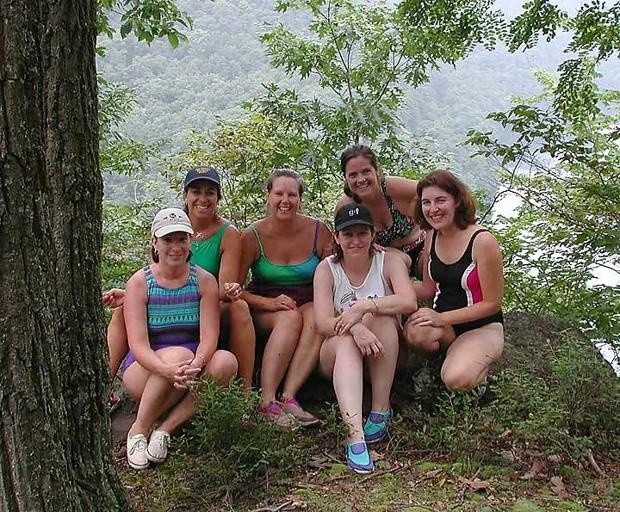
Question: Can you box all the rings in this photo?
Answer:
[236,289,238,294]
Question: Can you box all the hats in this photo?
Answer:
[184,165,220,191]
[151,208,194,239]
[334,203,374,233]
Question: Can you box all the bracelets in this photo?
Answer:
[197,352,207,365]
[368,294,379,317]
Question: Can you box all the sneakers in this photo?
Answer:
[256,401,299,431]
[126,423,149,470]
[279,398,319,427]
[345,429,375,474]
[144,426,171,463]
[363,407,393,443]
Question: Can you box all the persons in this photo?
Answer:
[313,203,417,475]
[124,208,239,469]
[334,145,423,282]
[239,169,334,433]
[404,170,504,405]
[101,166,255,415]
